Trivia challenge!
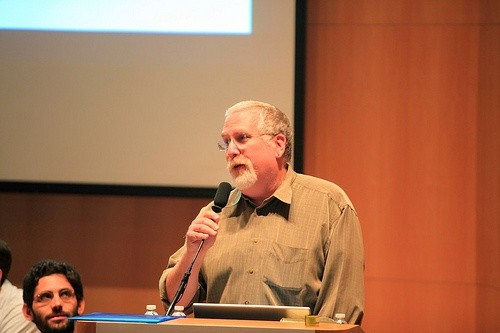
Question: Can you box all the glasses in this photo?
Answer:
[217,131,275,151]
[33,289,76,303]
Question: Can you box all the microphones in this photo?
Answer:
[165,182,232,316]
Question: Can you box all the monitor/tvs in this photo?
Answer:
[193,303,310,321]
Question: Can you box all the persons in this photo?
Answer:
[159,100,365,327]
[22,260,86,333]
[0,240,42,333]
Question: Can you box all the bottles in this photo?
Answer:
[334,314,347,324]
[144,304,158,315]
[172,306,186,316]
